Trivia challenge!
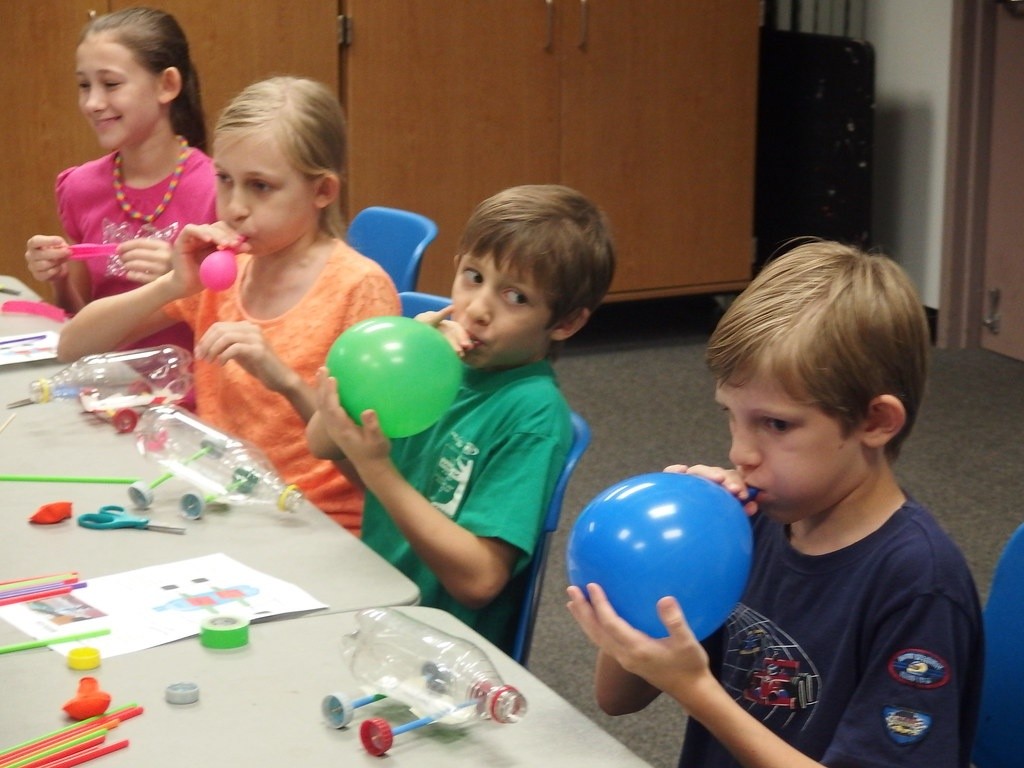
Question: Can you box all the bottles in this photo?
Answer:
[133,403,304,514]
[338,606,529,723]
[29,344,196,411]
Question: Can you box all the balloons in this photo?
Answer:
[568,473,760,643]
[65,244,115,259]
[325,315,464,438]
[198,232,246,291]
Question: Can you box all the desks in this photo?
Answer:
[1,273,656,767]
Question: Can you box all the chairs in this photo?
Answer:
[346,204,594,666]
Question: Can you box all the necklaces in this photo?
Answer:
[113,133,189,223]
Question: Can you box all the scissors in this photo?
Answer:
[5,386,79,410]
[77,504,187,536]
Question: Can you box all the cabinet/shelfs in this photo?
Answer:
[1,1,760,322]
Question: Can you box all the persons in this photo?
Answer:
[57,77,402,538]
[566,233,984,768]
[23,8,217,409]
[305,185,616,644]
